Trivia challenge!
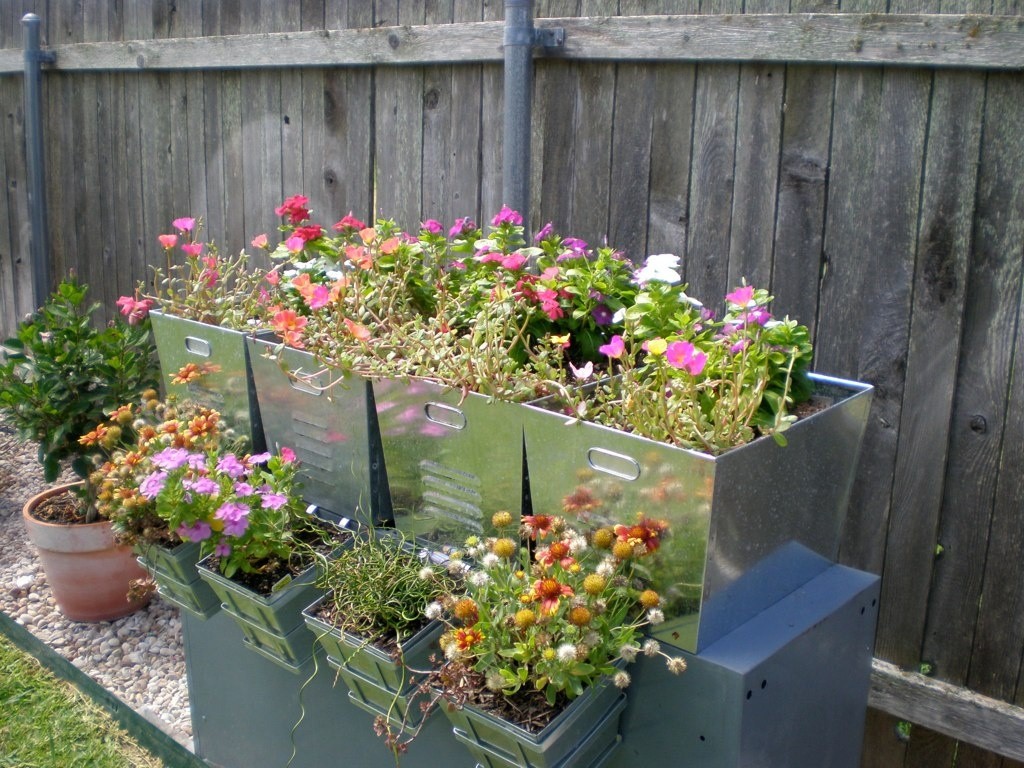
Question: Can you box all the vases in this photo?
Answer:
[136,529,222,621]
[195,505,358,674]
[147,299,873,655]
[23,478,151,623]
[428,646,628,768]
[302,546,470,737]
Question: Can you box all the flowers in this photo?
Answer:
[577,453,713,620]
[198,361,247,439]
[116,195,815,458]
[0,267,161,524]
[76,363,686,768]
